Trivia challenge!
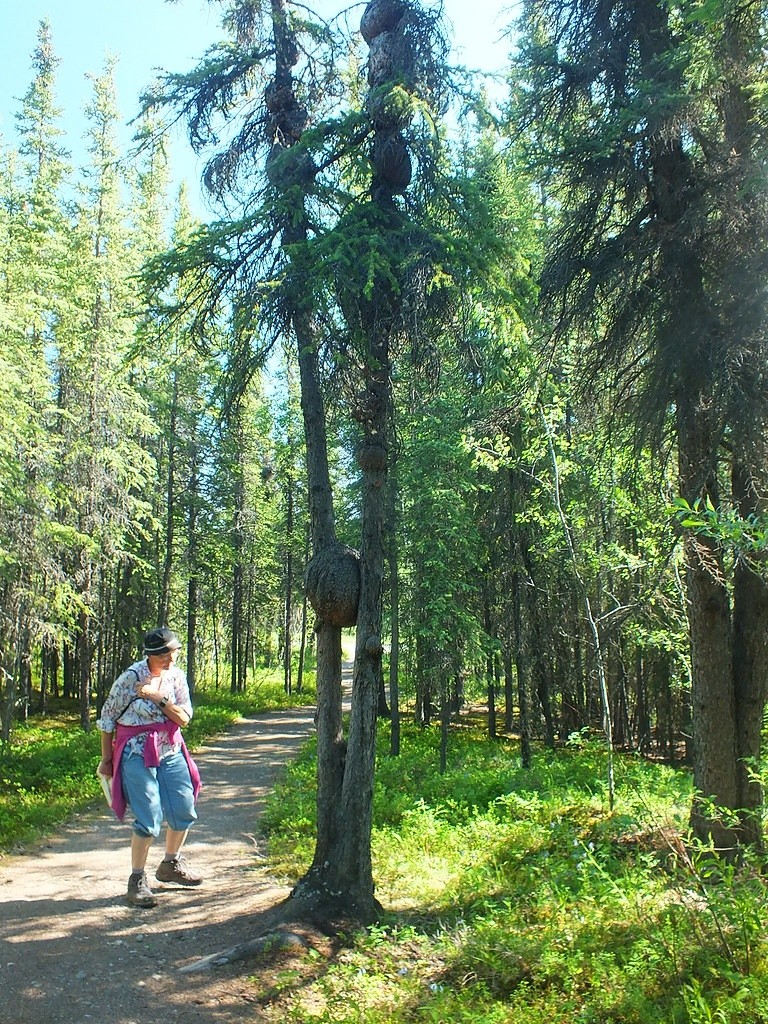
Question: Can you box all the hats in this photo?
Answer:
[143,628,183,655]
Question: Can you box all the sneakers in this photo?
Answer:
[128,873,158,907]
[155,855,203,886]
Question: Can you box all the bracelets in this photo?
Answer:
[157,696,169,709]
[101,758,112,764]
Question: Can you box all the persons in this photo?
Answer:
[98,629,203,908]
